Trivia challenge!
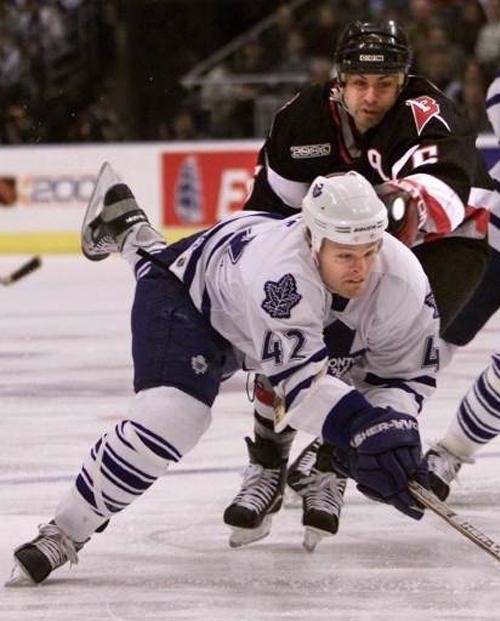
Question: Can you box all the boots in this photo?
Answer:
[13,519,89,585]
[223,436,349,535]
[357,443,463,521]
[81,183,148,261]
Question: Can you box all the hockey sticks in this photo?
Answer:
[1,256,42,284]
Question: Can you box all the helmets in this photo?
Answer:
[335,19,411,75]
[301,170,388,253]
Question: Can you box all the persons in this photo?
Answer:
[7,161,440,585]
[0,0,500,147]
[416,73,500,502]
[224,19,485,552]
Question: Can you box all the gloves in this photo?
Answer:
[373,179,427,249]
[321,389,429,520]
